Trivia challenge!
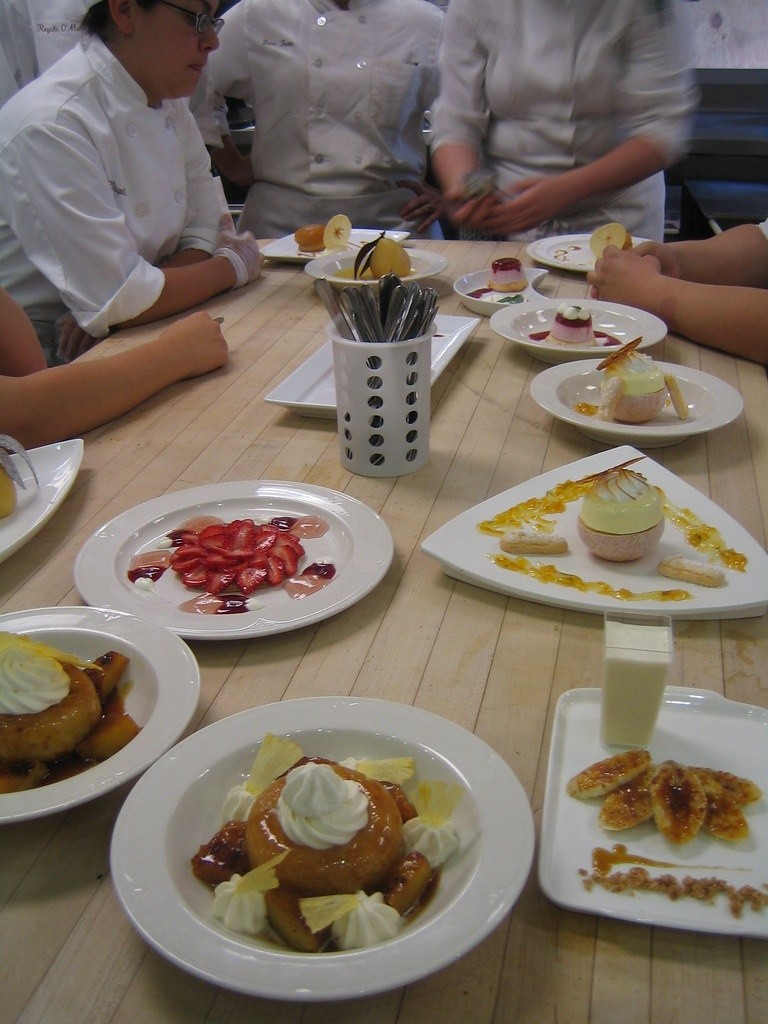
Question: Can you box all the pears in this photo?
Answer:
[370,233,411,279]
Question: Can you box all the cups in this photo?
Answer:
[596,607,675,753]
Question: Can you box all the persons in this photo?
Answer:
[188,0,448,239]
[0,0,263,369]
[586,219,768,365]
[430,0,694,240]
[0,287,229,454]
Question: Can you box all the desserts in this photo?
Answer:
[577,469,666,562]
[488,258,528,291]
[1,627,99,765]
[589,223,633,256]
[191,731,457,953]
[545,302,599,347]
[0,434,38,519]
[596,335,689,422]
[291,212,352,253]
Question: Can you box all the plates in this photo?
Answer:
[529,358,744,449]
[0,604,202,825]
[489,298,668,366]
[419,443,768,623]
[525,232,653,273]
[1,439,86,567]
[265,314,481,421]
[110,695,535,1003]
[258,228,412,264]
[72,480,394,642]
[538,684,768,942]
[304,248,450,299]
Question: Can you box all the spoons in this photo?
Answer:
[312,273,445,466]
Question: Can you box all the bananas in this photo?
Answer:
[566,749,764,846]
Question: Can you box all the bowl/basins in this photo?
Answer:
[452,267,550,317]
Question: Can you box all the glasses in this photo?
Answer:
[164,0,224,34]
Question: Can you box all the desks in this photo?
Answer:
[0,227,767,1023]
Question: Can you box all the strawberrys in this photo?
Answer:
[168,517,308,594]
